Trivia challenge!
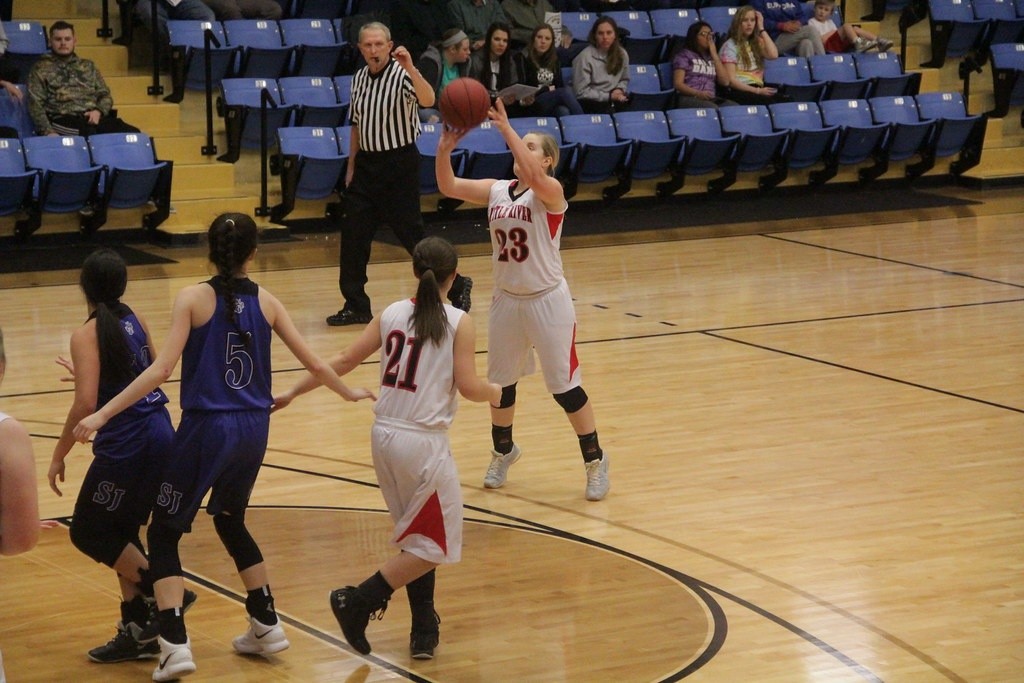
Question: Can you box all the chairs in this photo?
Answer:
[20,136,109,235]
[0,138,37,234]
[0,84,43,138]
[2,22,50,55]
[88,0,988,225]
[971,0,1024,51]
[982,43,1024,128]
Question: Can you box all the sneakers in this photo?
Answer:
[855,38,879,52]
[584,450,609,501]
[137,587,198,642]
[232,617,290,654]
[326,308,373,325]
[451,276,473,313]
[152,635,196,681]
[484,442,521,489]
[88,622,161,663]
[409,625,439,659]
[328,585,376,656]
[876,37,893,52]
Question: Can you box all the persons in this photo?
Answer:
[671,21,740,108]
[435,96,610,500]
[326,22,472,326]
[571,16,630,114]
[807,0,894,53]
[270,236,503,660]
[513,24,584,118]
[0,327,59,556]
[47,248,197,664]
[27,21,175,212]
[751,0,826,58]
[72,212,379,683]
[416,28,481,124]
[132,0,283,74]
[338,0,572,76]
[478,21,533,119]
[719,5,792,106]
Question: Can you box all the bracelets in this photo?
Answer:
[759,29,766,34]
[756,87,760,94]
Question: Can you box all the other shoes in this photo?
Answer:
[150,200,175,213]
[80,208,93,216]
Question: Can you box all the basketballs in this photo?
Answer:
[439,77,489,129]
